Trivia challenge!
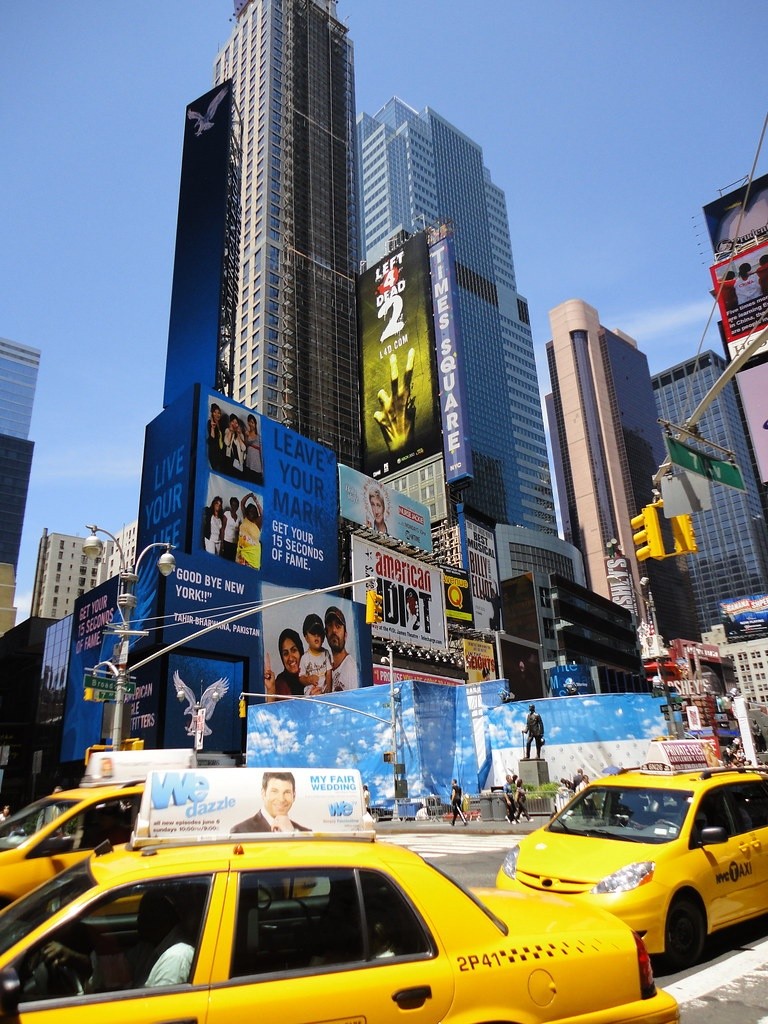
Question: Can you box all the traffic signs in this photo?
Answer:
[659,703,683,713]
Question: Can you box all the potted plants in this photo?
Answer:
[511,781,562,817]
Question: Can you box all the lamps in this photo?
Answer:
[425,649,434,660]
[406,644,416,657]
[434,650,443,662]
[441,652,450,663]
[416,647,427,657]
[384,638,397,648]
[457,655,464,666]
[449,655,457,665]
[397,641,410,653]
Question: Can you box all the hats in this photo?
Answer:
[325,606,345,626]
[302,613,324,636]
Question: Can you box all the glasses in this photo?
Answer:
[310,628,322,636]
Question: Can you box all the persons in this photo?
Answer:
[299,614,333,695]
[503,774,534,823]
[481,588,502,630]
[363,785,370,811]
[324,606,358,692]
[721,736,765,769]
[202,493,263,571]
[42,888,196,991]
[448,778,468,827]
[751,719,766,752]
[718,254,768,310]
[364,490,389,534]
[523,704,545,758]
[264,628,306,703]
[35,784,67,836]
[0,805,13,838]
[229,770,313,834]
[560,769,600,819]
[208,403,265,488]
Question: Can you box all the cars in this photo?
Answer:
[0,765,682,1024]
[0,747,318,920]
[492,737,768,975]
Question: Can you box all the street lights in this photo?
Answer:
[606,574,679,740]
[380,649,400,815]
[81,523,178,751]
[175,684,220,754]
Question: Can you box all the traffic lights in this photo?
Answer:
[383,752,392,762]
[238,700,246,718]
[84,746,106,766]
[365,589,383,624]
[630,506,666,562]
[121,740,145,751]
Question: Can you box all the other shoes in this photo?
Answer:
[504,814,534,826]
[464,822,468,826]
[449,821,454,825]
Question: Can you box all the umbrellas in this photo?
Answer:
[602,765,620,775]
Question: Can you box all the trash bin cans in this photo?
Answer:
[397,802,422,821]
[491,796,509,820]
[480,797,493,821]
